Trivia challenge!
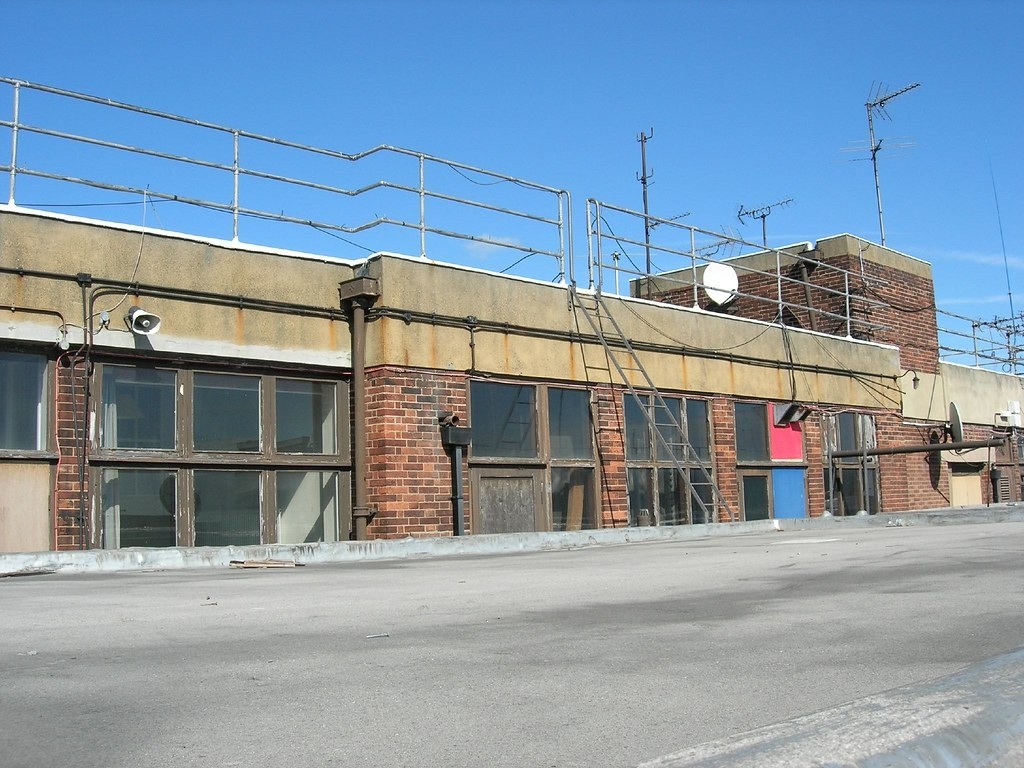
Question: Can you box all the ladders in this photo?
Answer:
[571,287,736,523]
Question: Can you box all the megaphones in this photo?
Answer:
[129,307,161,335]
